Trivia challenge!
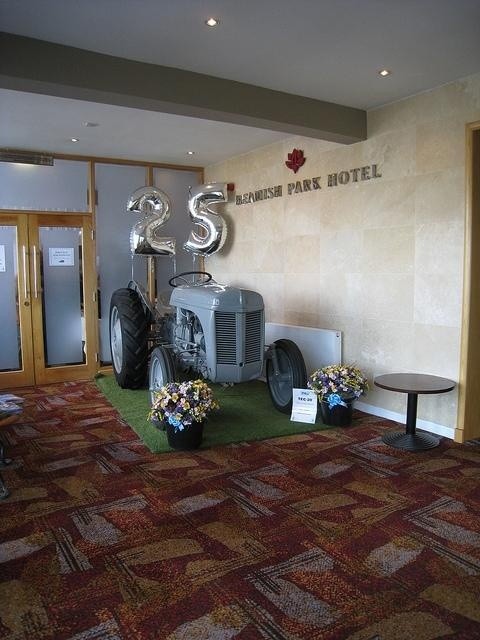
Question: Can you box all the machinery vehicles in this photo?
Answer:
[107,267,310,431]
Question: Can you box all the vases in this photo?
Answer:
[318,396,356,425]
[164,419,204,449]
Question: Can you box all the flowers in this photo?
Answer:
[147,378,222,433]
[305,361,370,410]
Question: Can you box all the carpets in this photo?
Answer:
[95,368,361,453]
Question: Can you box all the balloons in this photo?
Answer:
[127,185,175,256]
[182,183,228,258]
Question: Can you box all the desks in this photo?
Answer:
[373,372,457,452]
